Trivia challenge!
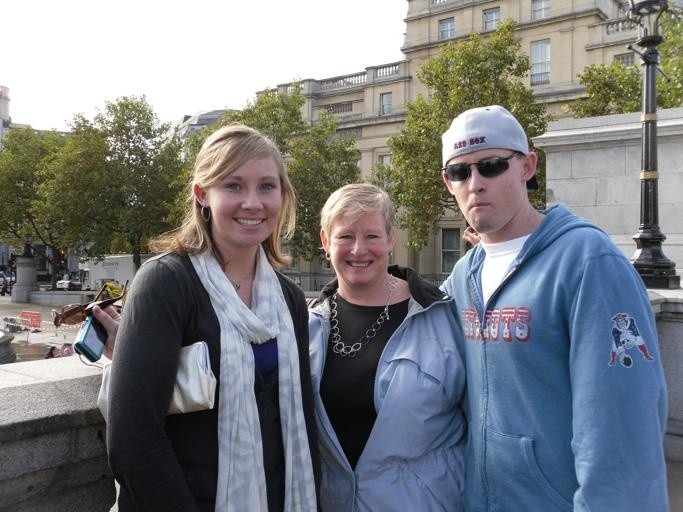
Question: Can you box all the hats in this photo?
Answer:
[440,105,529,170]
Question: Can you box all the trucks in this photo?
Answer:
[78,253,157,292]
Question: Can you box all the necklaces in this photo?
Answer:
[328,274,397,357]
[230,273,249,292]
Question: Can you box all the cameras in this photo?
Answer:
[73,315,108,362]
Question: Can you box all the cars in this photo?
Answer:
[0,270,15,287]
[55,277,81,291]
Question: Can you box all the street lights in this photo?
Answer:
[624,0,683,290]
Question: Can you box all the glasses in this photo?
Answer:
[440,151,517,182]
[50,280,129,327]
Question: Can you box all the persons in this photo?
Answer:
[81,183,466,512]
[101,123,320,511]
[436,104,668,511]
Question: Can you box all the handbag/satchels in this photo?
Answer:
[95,341,217,424]
